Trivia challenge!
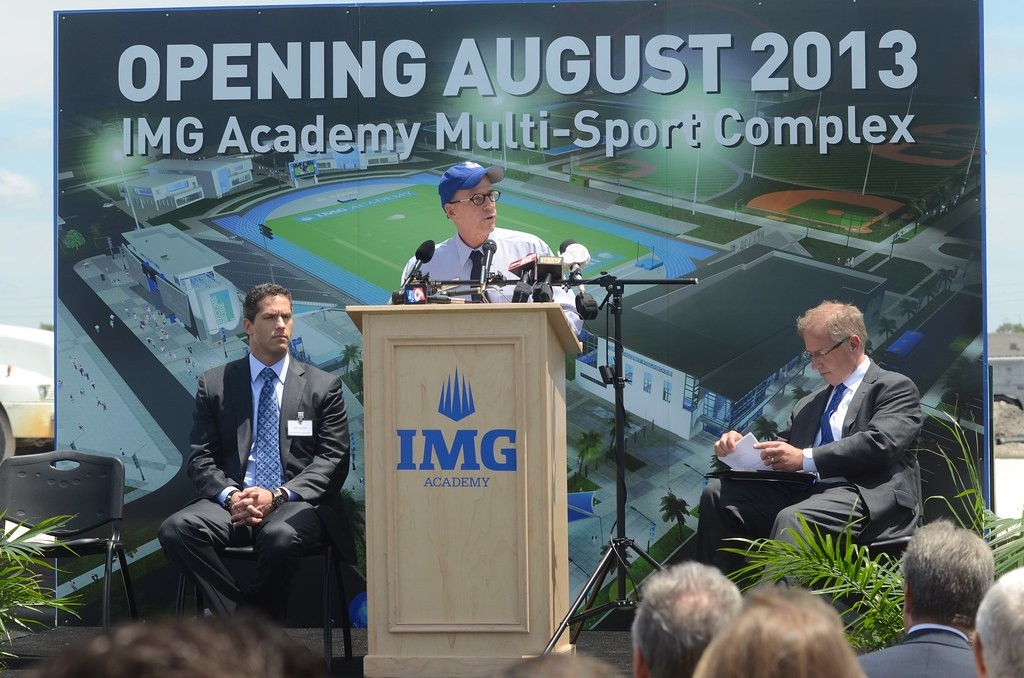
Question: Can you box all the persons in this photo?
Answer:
[631,560,743,678]
[696,301,923,589]
[857,520,995,678]
[974,566,1024,678]
[693,581,867,678]
[158,283,351,617]
[401,161,584,335]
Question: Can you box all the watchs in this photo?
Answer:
[270,489,285,508]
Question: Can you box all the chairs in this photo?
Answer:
[737,458,923,551]
[0,451,138,661]
[175,497,358,678]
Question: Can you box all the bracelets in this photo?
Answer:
[224,490,237,511]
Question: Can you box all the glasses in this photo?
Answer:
[800,335,852,360]
[448,190,501,205]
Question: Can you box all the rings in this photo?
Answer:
[772,458,774,463]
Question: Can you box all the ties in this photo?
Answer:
[820,382,846,446]
[469,250,484,302]
[253,369,280,490]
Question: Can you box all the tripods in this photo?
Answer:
[429,277,698,656]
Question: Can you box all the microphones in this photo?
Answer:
[392,240,598,320]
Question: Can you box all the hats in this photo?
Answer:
[438,161,505,206]
